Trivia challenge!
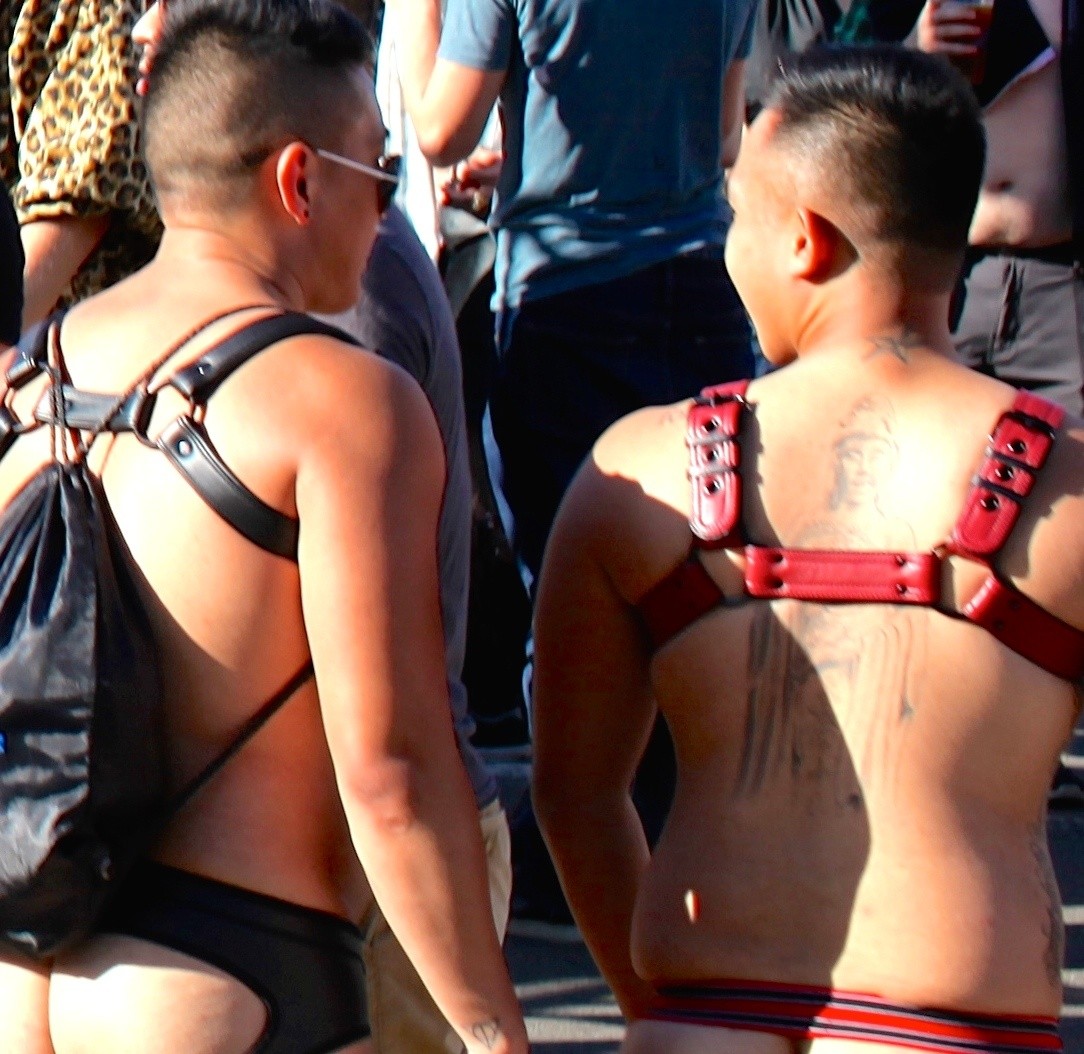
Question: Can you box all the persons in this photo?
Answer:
[0,0,537,1054]
[0,3,1084,918]
[540,51,1084,1054]
[127,0,515,1054]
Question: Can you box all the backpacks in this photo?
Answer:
[0,301,366,969]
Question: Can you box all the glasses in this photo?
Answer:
[244,137,398,211]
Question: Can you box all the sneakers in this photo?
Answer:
[468,701,534,760]
